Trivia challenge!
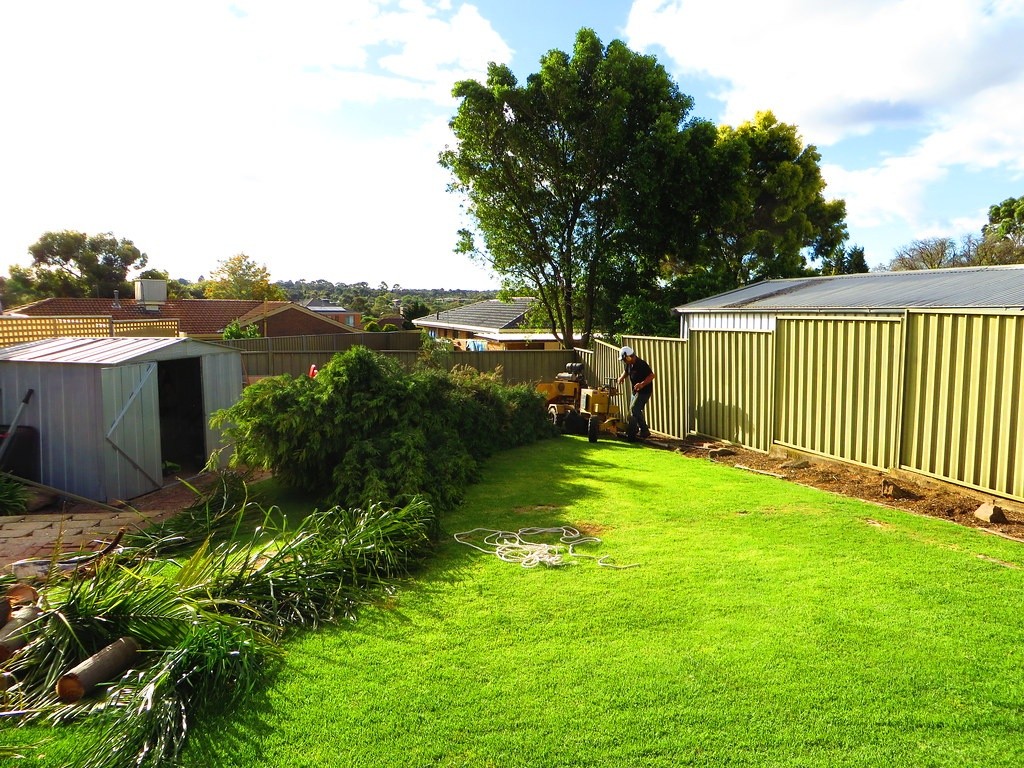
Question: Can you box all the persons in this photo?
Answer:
[615,346,656,445]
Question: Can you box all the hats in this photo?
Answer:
[618,346,633,361]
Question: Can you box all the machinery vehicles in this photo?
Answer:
[532,362,633,443]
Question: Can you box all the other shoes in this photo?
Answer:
[636,431,650,437]
